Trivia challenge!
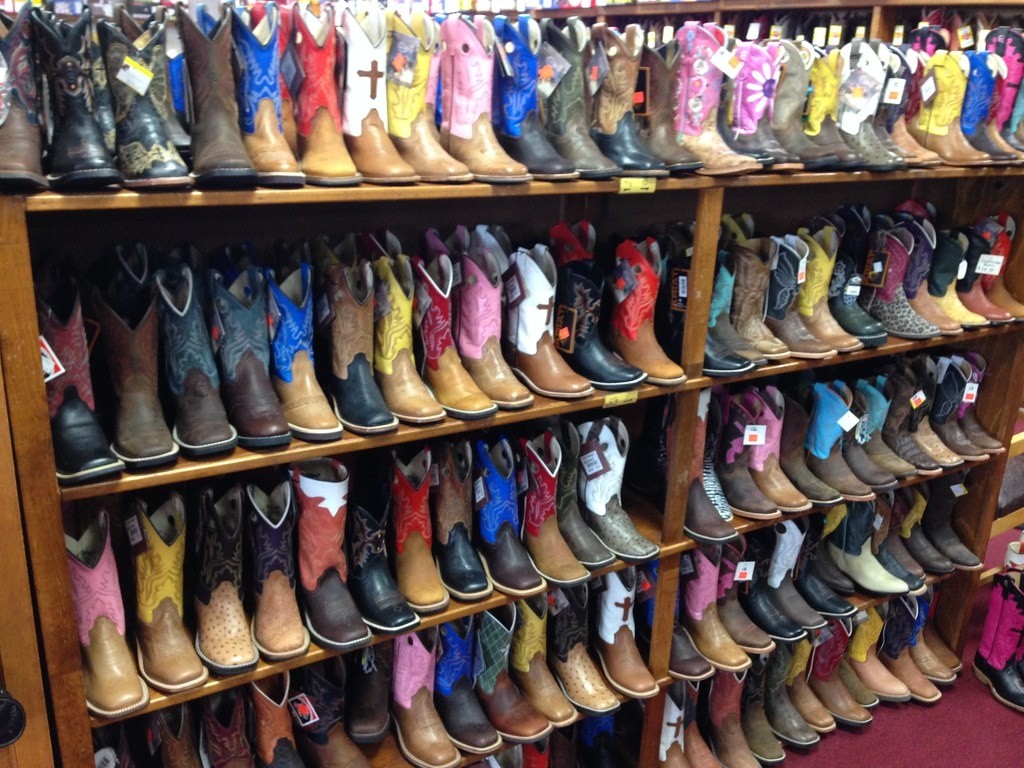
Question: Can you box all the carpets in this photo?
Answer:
[769,524,1024,768]
[1012,417,1023,435]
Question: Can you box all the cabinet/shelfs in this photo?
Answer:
[0,169,1024,768]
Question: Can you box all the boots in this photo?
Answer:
[1,0,1024,768]
